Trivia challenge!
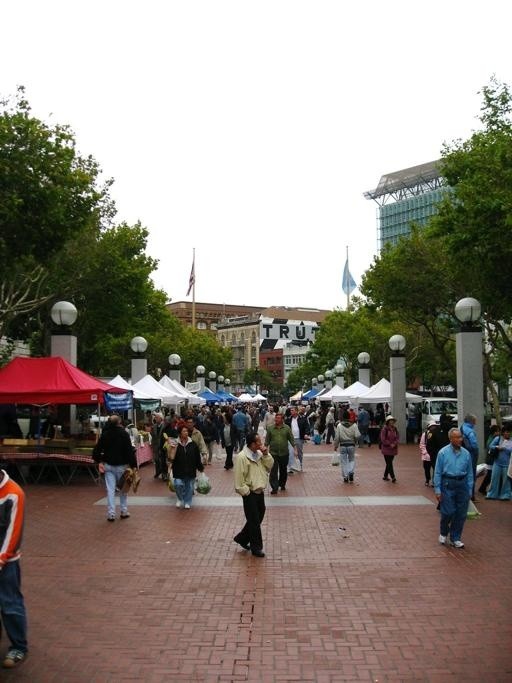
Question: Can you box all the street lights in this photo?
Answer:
[334,364,345,388]
[254,366,262,394]
[195,363,206,387]
[271,369,278,394]
[454,296,485,463]
[167,352,182,384]
[208,370,217,394]
[51,300,80,435]
[357,351,371,387]
[311,376,318,389]
[130,335,149,385]
[317,373,325,388]
[225,378,232,393]
[217,374,225,392]
[388,333,407,444]
[324,369,333,388]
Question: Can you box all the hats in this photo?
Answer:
[386,415,396,422]
[427,420,440,428]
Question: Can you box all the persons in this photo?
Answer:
[234,431,276,557]
[42,414,54,438]
[93,400,277,522]
[434,427,475,547]
[405,401,511,511]
[262,402,402,495]
[0,467,29,668]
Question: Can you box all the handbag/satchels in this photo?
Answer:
[485,450,496,464]
[461,434,471,450]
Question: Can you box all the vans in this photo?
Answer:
[406,396,457,444]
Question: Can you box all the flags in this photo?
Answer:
[342,260,357,294]
[186,261,195,294]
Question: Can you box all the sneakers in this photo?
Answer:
[106,515,115,520]
[176,499,181,508]
[252,550,264,557]
[184,504,190,509]
[271,490,278,495]
[450,541,464,549]
[2,650,24,667]
[120,511,130,517]
[350,473,353,482]
[234,537,249,550]
[439,535,446,544]
